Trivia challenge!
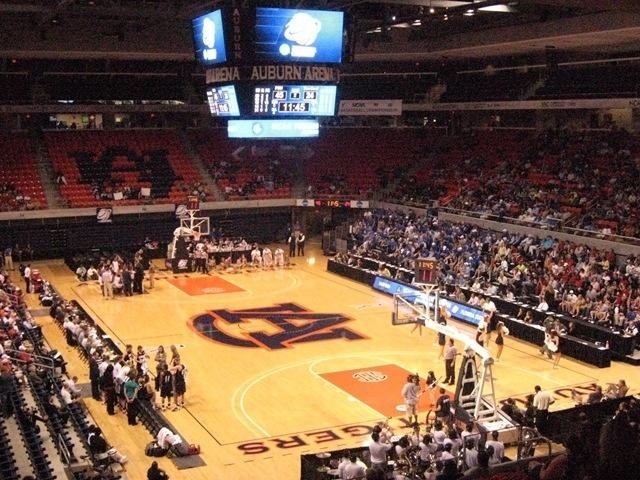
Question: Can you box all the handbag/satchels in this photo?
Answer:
[145,438,199,457]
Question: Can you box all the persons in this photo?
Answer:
[1,240,53,306]
[57,158,305,299]
[339,371,640,479]
[2,179,36,211]
[314,109,640,369]
[440,338,457,385]
[56,115,199,131]
[1,299,187,479]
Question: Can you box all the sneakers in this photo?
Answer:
[158,403,184,411]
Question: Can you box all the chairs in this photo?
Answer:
[1,360,125,480]
